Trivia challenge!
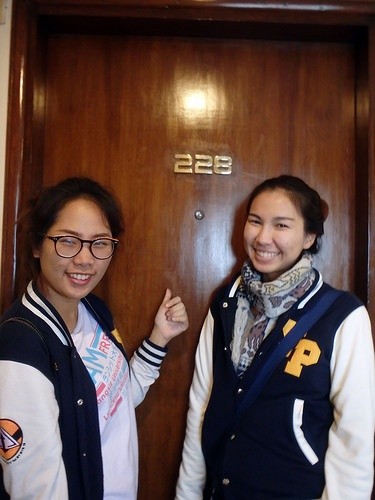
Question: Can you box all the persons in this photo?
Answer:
[0,177,188,500]
[175,176,375,500]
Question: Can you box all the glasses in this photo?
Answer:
[38,231,119,259]
